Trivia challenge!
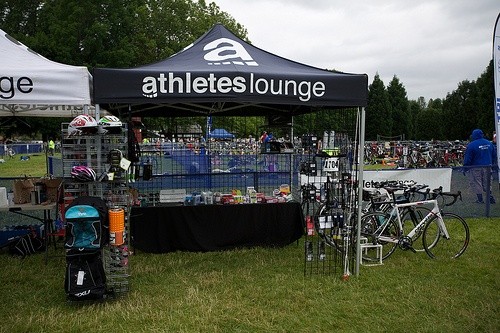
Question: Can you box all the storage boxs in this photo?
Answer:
[0,222,44,246]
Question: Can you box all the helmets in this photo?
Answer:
[97,115,123,136]
[67,114,98,138]
[71,166,96,182]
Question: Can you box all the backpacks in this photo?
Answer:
[65,251,108,302]
[63,196,110,252]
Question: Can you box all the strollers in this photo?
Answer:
[63,195,110,302]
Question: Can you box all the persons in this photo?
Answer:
[461,128,500,205]
[260,130,277,154]
[48,136,57,157]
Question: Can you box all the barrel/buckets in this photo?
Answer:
[108,209,125,246]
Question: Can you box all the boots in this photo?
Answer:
[472,194,484,204]
[490,195,496,203]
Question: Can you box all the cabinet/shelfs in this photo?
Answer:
[60,121,132,294]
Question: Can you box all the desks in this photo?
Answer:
[0,202,56,265]
[131,202,303,255]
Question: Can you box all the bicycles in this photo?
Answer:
[3,146,15,163]
[311,178,472,267]
[139,137,466,167]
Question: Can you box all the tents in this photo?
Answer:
[0,20,369,285]
